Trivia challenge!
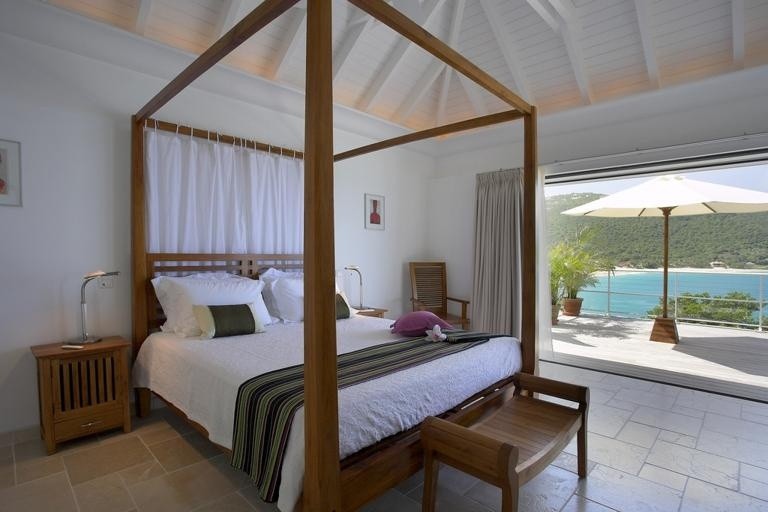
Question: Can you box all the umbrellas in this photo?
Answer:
[560,174,768,317]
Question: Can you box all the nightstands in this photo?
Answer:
[30,336,133,456]
[354,307,388,318]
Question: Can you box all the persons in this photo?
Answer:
[369,200,380,224]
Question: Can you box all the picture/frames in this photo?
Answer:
[365,193,385,230]
[0,138,22,208]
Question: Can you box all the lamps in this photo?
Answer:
[345,265,367,310]
[64,270,121,344]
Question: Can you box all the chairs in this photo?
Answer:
[409,262,470,330]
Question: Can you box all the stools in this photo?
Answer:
[419,373,591,511]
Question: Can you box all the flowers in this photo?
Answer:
[424,324,447,342]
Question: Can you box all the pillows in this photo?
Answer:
[150,268,359,340]
[390,311,453,335]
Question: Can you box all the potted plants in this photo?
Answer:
[551,223,615,326]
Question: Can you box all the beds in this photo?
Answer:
[147,253,522,511]
